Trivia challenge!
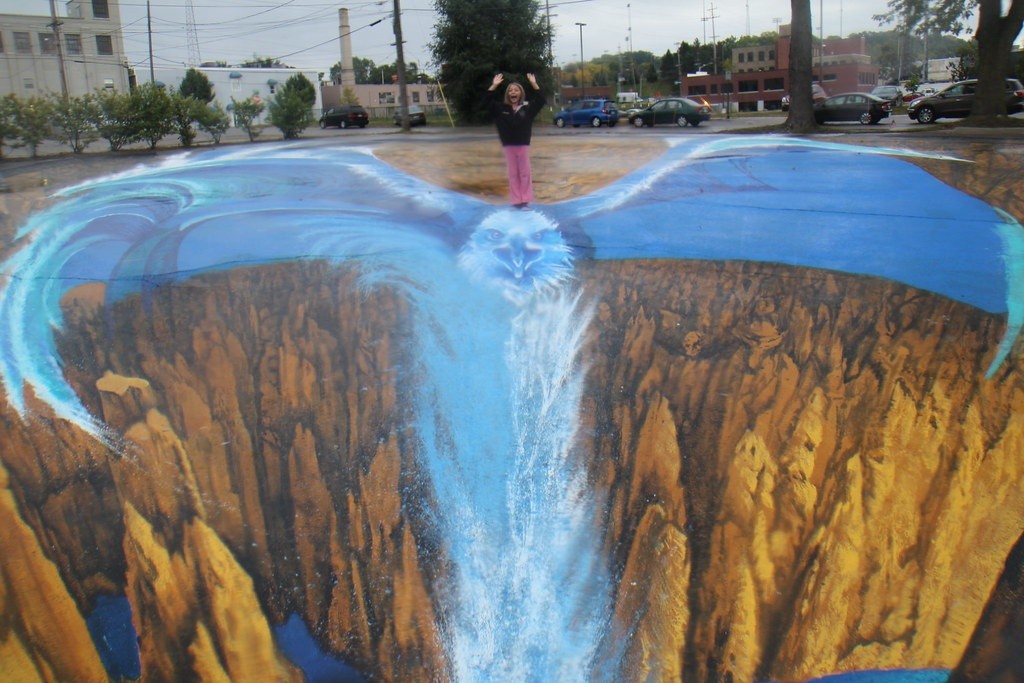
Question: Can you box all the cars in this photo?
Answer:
[815,92,893,125]
[902,88,934,102]
[391,105,426,126]
[318,105,370,129]
[628,98,711,128]
[872,86,903,107]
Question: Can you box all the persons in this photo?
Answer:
[482,73,546,206]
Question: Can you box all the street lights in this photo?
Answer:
[575,22,587,98]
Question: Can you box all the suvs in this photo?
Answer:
[906,77,1024,124]
[552,98,620,127]
